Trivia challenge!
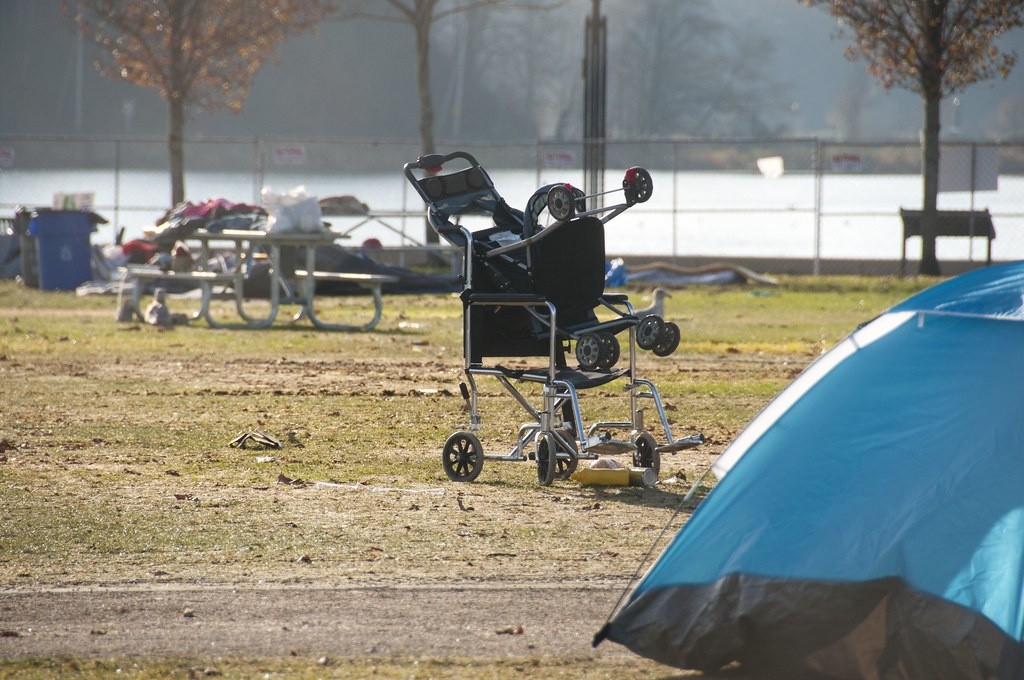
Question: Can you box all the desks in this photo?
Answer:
[184,227,351,329]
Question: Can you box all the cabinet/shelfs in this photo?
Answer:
[322,210,462,278]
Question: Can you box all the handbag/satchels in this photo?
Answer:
[259,186,327,238]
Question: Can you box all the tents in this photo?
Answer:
[591,261,1024,680]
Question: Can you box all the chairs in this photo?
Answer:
[126,267,237,321]
[266,271,400,330]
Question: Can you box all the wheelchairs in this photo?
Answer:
[436,219,663,486]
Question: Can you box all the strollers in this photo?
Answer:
[401,150,681,369]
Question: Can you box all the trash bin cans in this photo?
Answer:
[16,208,99,290]
